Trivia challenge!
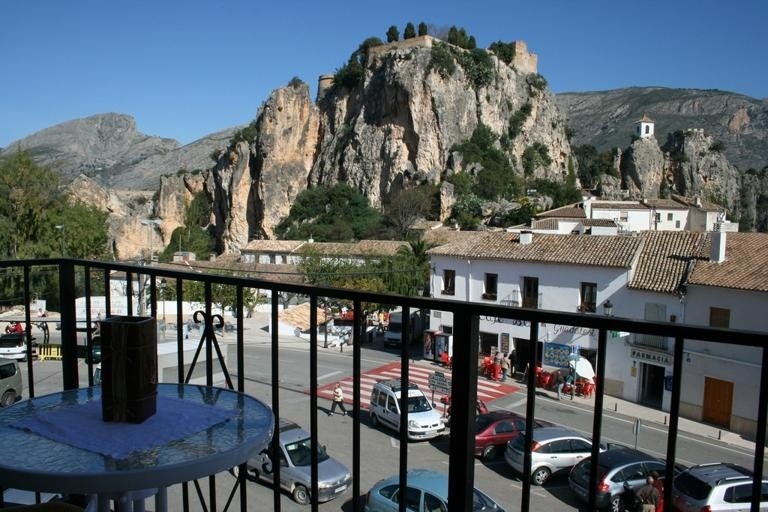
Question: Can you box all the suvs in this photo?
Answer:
[370,380,446,440]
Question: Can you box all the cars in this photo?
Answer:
[475,410,767,511]
[230,419,352,505]
[0,331,28,408]
[86,336,102,363]
[365,468,505,512]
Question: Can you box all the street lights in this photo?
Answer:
[141,216,161,257]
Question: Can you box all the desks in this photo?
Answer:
[0,379,279,511]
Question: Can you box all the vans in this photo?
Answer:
[383,309,422,349]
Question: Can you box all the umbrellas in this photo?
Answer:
[568,353,595,378]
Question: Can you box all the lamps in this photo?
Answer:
[603,299,614,317]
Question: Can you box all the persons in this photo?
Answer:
[636,471,665,512]
[37,309,48,317]
[424,335,432,354]
[372,309,389,333]
[557,369,595,400]
[329,383,349,415]
[6,321,22,333]
[493,350,517,382]
